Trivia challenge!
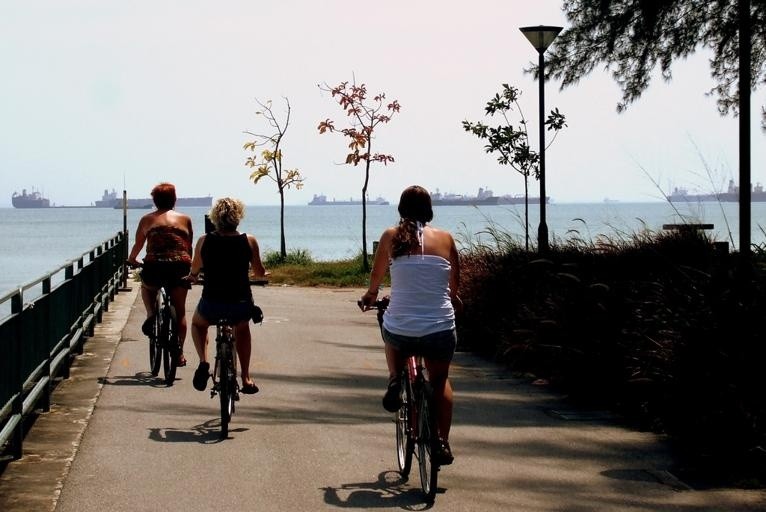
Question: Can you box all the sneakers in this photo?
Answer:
[141,313,186,367]
[382,375,454,466]
[192,359,260,394]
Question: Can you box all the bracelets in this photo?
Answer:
[190,272,199,277]
[368,289,378,295]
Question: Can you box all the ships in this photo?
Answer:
[95,188,212,207]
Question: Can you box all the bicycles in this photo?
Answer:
[123,259,194,386]
[179,277,270,439]
[357,297,474,505]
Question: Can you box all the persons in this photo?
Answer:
[125,184,193,366]
[184,197,272,393]
[361,185,459,465]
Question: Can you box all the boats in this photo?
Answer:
[11,186,49,209]
[113,203,153,209]
